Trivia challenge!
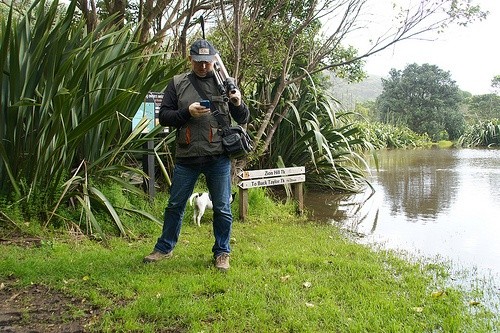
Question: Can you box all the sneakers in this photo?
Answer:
[143,248,174,262]
[213,252,230,270]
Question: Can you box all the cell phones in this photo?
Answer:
[200,100,210,109]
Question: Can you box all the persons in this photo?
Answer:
[143,39,253,270]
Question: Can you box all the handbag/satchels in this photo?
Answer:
[222,125,255,161]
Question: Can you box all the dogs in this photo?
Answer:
[190,191,237,227]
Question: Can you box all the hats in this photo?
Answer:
[189,39,218,63]
